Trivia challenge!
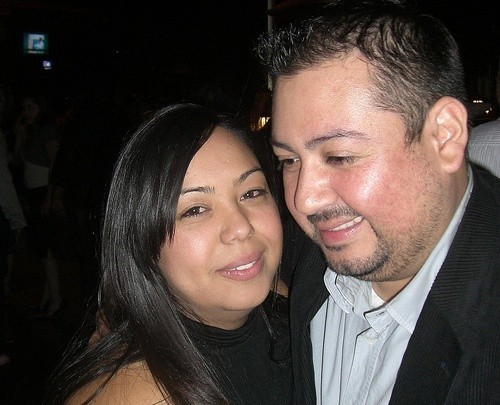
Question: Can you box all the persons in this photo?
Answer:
[47,106,299,403]
[12,94,67,311]
[464,72,499,179]
[268,0,500,405]
